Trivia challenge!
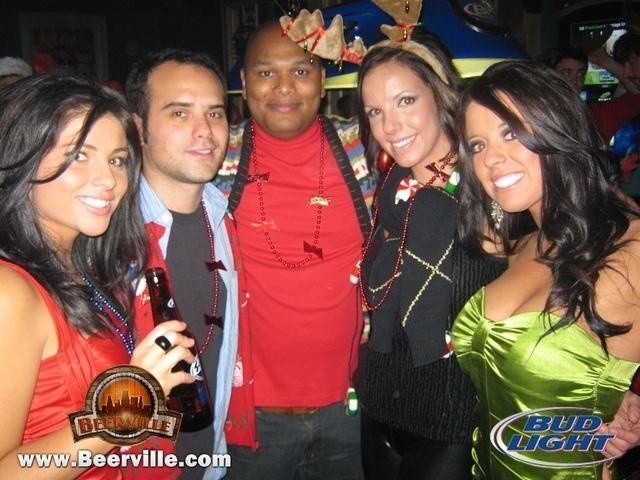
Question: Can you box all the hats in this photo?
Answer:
[0,57,33,77]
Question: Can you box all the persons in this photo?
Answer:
[0,0,639,480]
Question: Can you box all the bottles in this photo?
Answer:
[145,266,215,433]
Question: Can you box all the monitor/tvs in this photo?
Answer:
[570,17,630,88]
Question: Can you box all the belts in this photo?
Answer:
[255,406,319,414]
